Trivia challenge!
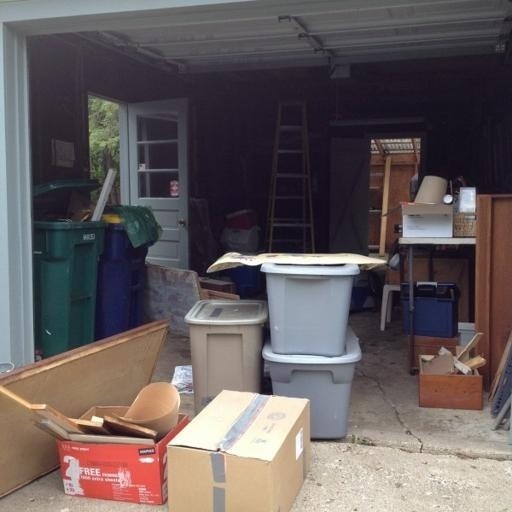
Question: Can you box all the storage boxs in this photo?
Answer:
[224,207,259,234]
[431,254,470,324]
[403,254,430,282]
[260,326,362,439]
[55,405,189,505]
[225,266,261,298]
[403,201,452,240]
[450,206,477,242]
[165,389,311,512]
[417,353,485,410]
[183,299,268,417]
[261,263,361,357]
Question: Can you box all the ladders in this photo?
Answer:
[367,155,392,256]
[264,97,316,254]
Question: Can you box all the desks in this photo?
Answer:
[398,190,477,375]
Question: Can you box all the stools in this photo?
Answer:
[376,280,404,334]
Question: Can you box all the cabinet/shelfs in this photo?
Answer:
[475,193,512,390]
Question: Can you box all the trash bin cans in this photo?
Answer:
[95,205,148,339]
[31,179,106,357]
